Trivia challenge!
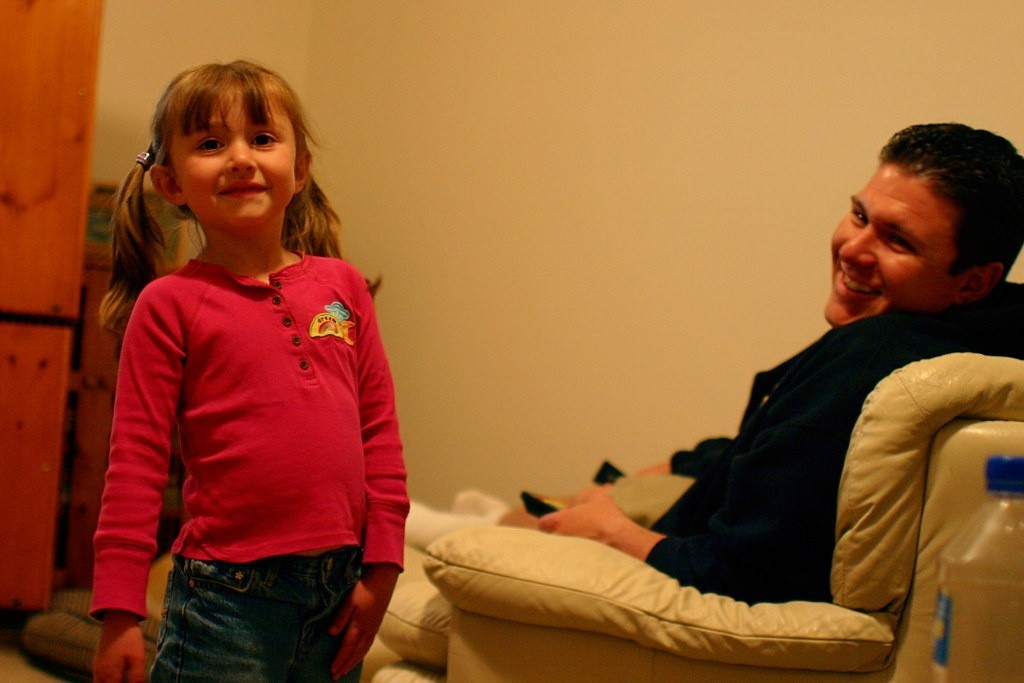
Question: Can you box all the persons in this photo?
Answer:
[404,124,1024,605]
[89,60,411,683]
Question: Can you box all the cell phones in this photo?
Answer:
[520,491,558,519]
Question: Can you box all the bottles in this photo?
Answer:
[929,456,1024,683]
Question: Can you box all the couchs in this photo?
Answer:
[376,350,1024,683]
